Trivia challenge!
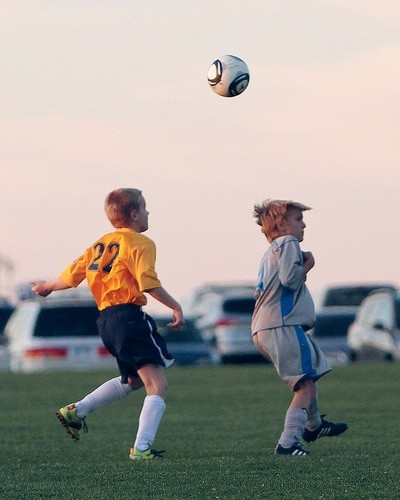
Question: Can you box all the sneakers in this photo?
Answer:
[57,402,88,441]
[128,444,166,461]
[274,442,311,456]
[301,414,349,441]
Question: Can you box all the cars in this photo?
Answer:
[152,318,219,366]
[313,306,359,360]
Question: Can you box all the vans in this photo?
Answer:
[324,287,399,322]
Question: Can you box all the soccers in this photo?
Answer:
[206,54,250,98]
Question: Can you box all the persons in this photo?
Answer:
[31,188,184,460]
[251,201,347,454]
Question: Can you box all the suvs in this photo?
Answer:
[193,287,269,363]
[4,297,118,371]
